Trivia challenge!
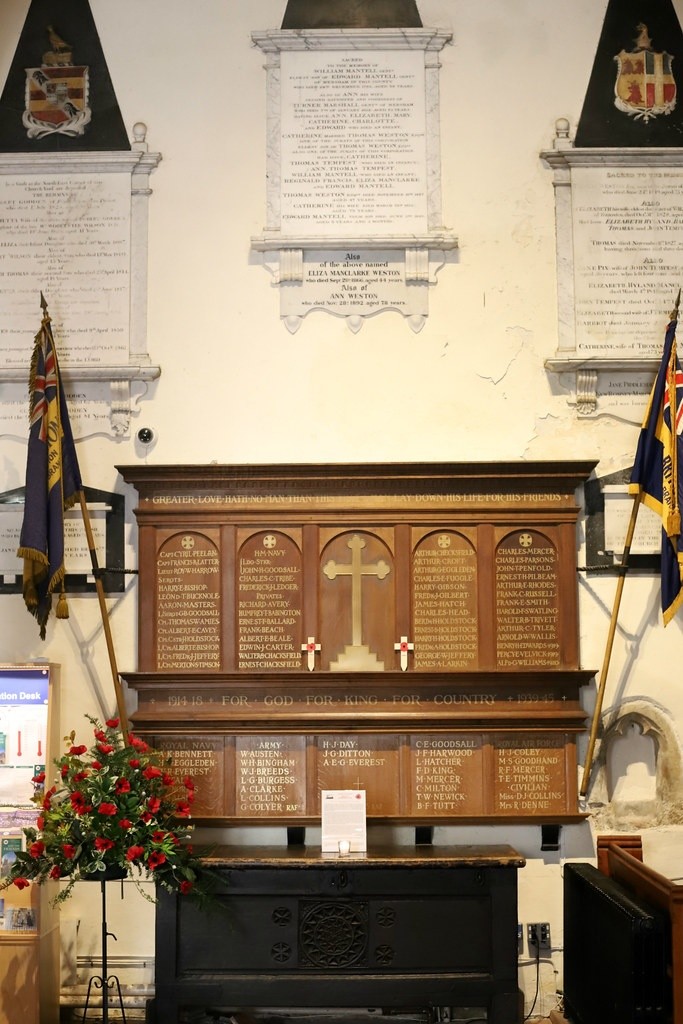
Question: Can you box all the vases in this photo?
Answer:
[76,848,129,881]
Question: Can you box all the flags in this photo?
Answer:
[17,321,82,640]
[628,318,683,629]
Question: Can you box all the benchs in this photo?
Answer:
[606,844,683,1024]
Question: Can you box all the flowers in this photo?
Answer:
[0,713,235,914]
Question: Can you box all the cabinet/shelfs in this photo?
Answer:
[0,833,62,1024]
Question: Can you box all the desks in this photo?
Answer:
[144,842,527,1024]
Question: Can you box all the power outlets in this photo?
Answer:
[527,924,551,942]
[518,924,523,940]
